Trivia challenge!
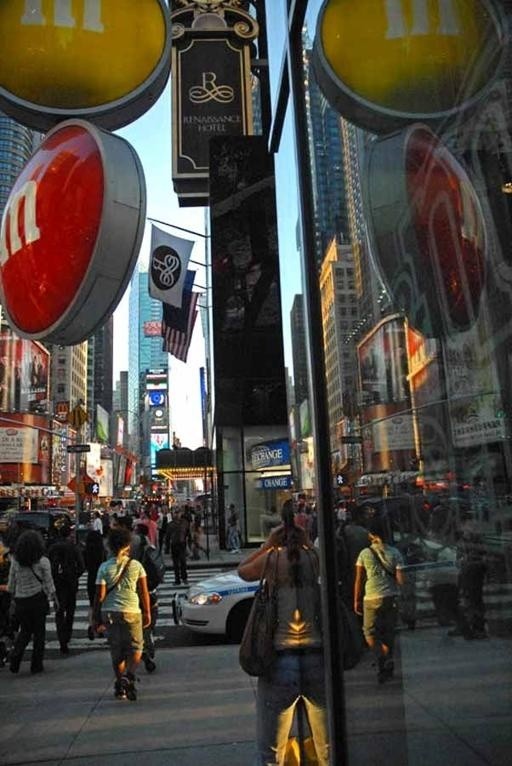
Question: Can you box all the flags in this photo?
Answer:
[145,221,201,364]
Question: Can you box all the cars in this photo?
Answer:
[390,530,460,632]
[171,569,265,644]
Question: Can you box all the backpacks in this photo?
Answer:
[49,544,76,584]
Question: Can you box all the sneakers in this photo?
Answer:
[10,625,155,700]
[377,657,394,683]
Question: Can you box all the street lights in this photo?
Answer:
[114,408,145,479]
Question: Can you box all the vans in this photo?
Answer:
[109,499,136,508]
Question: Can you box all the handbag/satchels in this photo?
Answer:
[142,543,167,591]
[240,580,278,677]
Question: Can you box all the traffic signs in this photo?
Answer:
[341,435,363,445]
[67,444,90,453]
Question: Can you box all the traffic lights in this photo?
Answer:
[85,483,100,496]
[431,200,472,334]
[335,472,350,486]
[491,397,505,419]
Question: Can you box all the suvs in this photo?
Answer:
[354,495,430,535]
[423,487,489,525]
[14,507,72,544]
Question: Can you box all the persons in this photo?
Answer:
[332,474,490,680]
[96,529,151,701]
[2,500,209,674]
[227,503,241,554]
[238,494,326,766]
